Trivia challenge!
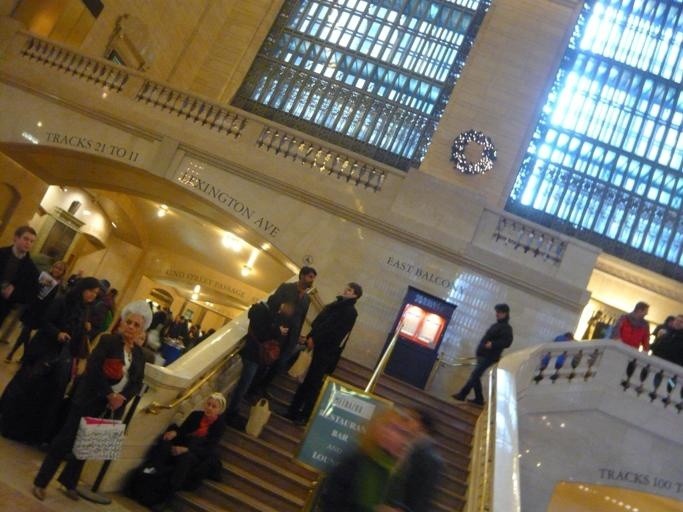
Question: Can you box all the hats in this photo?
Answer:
[99,279,110,292]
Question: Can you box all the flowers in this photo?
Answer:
[450,130,496,175]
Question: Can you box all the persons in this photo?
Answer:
[613,301,683,411]
[535,332,576,381]
[140,393,228,510]
[146,304,215,358]
[309,406,440,512]
[1,226,153,503]
[225,267,363,430]
[451,304,513,405]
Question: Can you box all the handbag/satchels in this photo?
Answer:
[288,347,313,384]
[102,358,123,380]
[245,399,271,437]
[72,402,126,460]
[259,340,281,364]
